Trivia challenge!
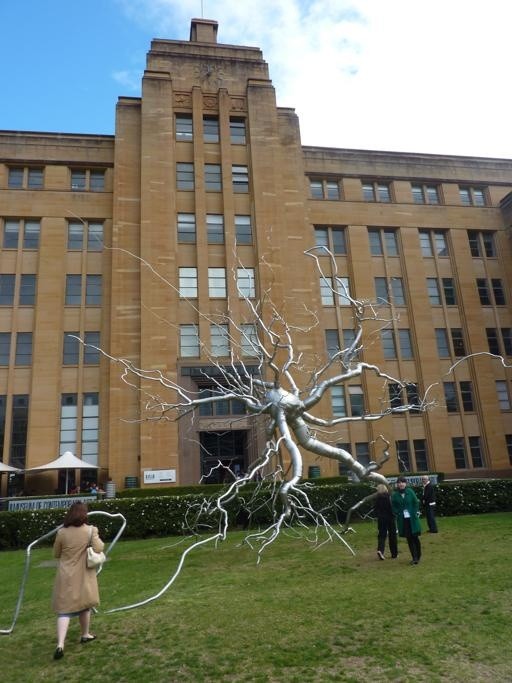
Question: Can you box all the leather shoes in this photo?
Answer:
[410,560,418,565]
[427,530,438,533]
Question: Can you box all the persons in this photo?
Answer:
[374,475,438,565]
[69,480,99,494]
[53,502,105,659]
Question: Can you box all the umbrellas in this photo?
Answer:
[24,450,101,494]
[0,462,24,472]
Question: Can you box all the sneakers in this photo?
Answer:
[377,550,398,560]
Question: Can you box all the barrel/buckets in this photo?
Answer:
[124,476,137,488]
[106,482,116,499]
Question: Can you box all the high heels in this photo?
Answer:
[53,647,64,660]
[81,634,97,643]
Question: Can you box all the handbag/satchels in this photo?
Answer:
[86,525,106,568]
